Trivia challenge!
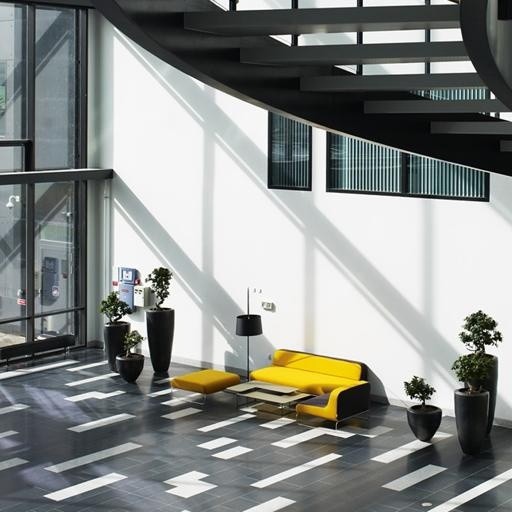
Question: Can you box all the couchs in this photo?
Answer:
[248,349,368,395]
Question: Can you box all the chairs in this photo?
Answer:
[294,381,371,429]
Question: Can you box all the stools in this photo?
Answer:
[171,368,241,404]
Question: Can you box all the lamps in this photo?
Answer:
[6,195,19,209]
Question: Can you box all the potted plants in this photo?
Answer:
[97,267,174,383]
[404,310,504,455]
